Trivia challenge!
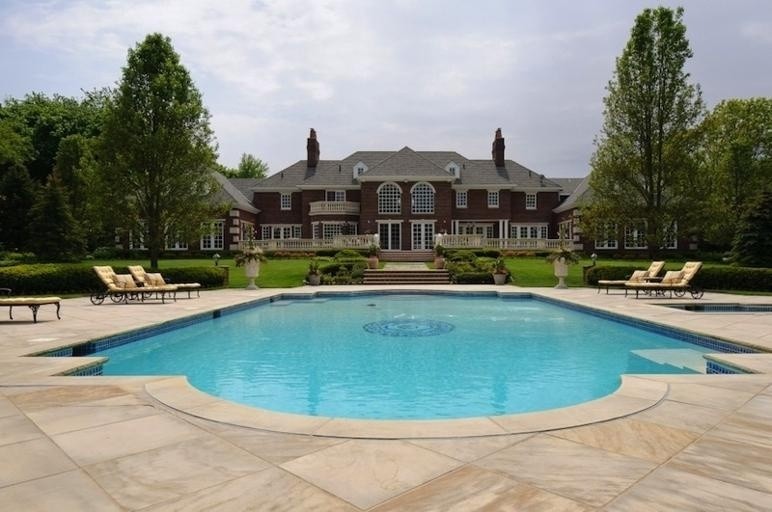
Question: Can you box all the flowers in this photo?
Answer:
[540,225,584,264]
[233,223,269,268]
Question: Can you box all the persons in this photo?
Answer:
[443,229,451,236]
[434,228,443,248]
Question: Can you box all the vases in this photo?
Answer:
[553,258,568,289]
[245,255,261,289]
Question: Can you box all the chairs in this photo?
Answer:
[597,261,668,295]
[129,264,201,299]
[90,264,178,304]
[626,260,706,298]
[1,285,62,326]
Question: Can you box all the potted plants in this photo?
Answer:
[308,257,322,285]
[367,243,379,269]
[490,250,511,285]
[434,244,446,270]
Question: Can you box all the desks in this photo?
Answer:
[644,276,665,297]
[131,280,145,302]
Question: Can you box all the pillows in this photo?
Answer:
[630,268,650,282]
[660,270,684,283]
[144,271,166,286]
[111,272,138,289]
